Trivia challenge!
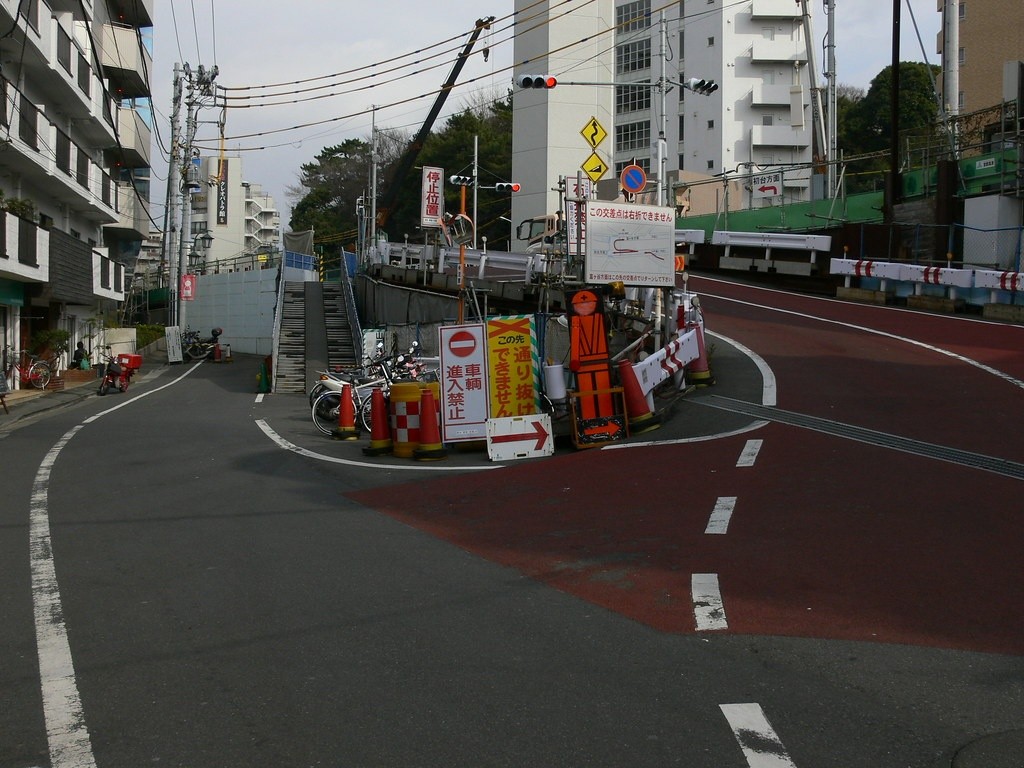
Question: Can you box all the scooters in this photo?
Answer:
[179,324,222,364]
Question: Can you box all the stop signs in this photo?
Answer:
[438,323,492,444]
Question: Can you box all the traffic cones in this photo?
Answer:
[330,384,360,442]
[411,389,448,461]
[255,362,271,394]
[688,322,716,388]
[617,358,660,434]
[362,388,394,458]
[214,345,223,363]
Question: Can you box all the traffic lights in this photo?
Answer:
[688,77,719,95]
[450,175,474,187]
[496,183,521,193]
[517,74,557,90]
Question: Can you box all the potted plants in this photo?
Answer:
[83,305,120,377]
[33,327,73,392]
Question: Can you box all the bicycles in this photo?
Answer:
[4,345,51,390]
[309,340,428,436]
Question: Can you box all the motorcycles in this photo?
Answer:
[98,343,144,396]
